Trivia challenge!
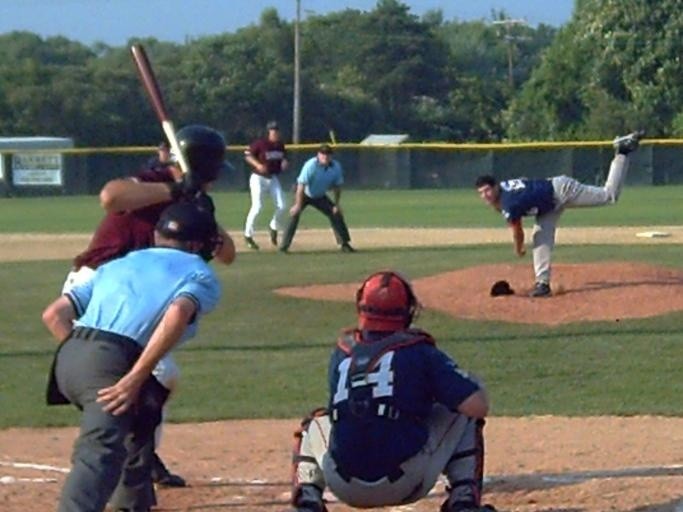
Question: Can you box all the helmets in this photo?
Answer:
[164,126,235,179]
[155,203,222,261]
[355,270,422,331]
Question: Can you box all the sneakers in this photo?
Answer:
[279,245,289,254]
[337,243,354,252]
[247,236,259,249]
[266,224,277,247]
[612,130,646,155]
[151,453,185,487]
[528,282,552,298]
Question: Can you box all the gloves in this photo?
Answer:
[164,171,214,214]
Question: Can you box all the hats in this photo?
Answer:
[318,144,333,154]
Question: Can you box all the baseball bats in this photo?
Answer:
[132,43,189,177]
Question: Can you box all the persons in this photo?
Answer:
[278,144,354,253]
[242,120,288,250]
[474,131,644,297]
[293,273,494,511]
[61,123,236,489]
[43,203,224,512]
